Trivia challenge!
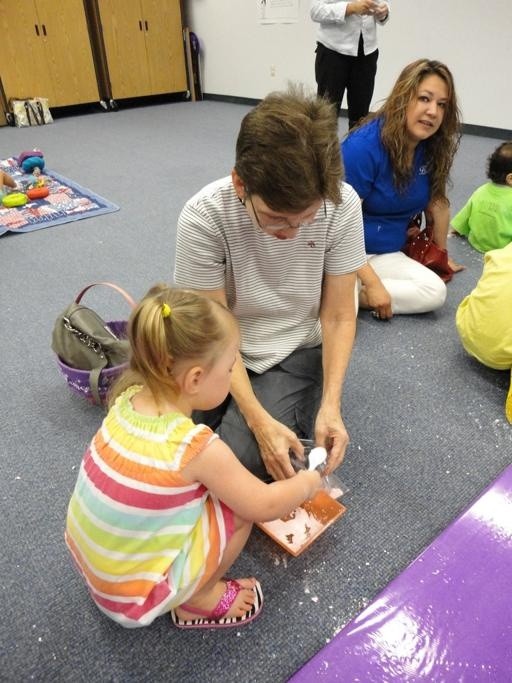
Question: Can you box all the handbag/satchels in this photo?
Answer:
[12,98,54,128]
[400,209,454,283]
[51,302,131,369]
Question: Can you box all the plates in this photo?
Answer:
[253,492,345,557]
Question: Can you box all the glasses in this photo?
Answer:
[245,177,329,231]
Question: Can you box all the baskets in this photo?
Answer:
[55,281,137,410]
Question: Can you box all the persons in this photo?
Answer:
[306,0,389,146]
[167,75,365,494]
[64,280,327,633]
[444,139,511,258]
[452,238,512,371]
[339,56,470,323]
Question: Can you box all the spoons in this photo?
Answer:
[305,446,329,471]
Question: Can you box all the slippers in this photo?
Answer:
[170,577,264,629]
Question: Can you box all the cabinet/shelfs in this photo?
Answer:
[90,0,191,111]
[0,0,110,126]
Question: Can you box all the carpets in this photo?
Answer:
[0,155,120,239]
